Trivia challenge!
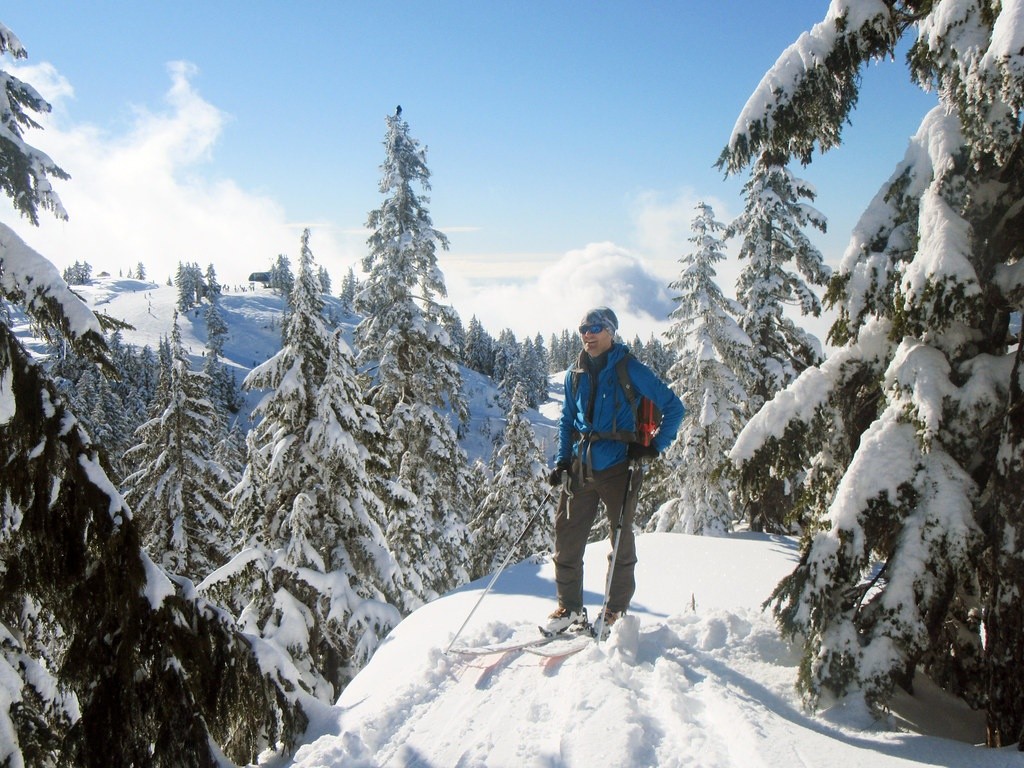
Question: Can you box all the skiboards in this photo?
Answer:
[448,605,618,659]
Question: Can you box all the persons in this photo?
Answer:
[539,306,686,641]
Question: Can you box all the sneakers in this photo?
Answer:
[543,607,583,632]
[593,604,621,635]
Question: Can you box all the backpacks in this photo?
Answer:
[573,354,662,487]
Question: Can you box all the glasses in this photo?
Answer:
[579,325,605,334]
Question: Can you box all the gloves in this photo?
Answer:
[547,461,570,486]
[628,440,659,459]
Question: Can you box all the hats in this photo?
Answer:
[584,305,619,335]
